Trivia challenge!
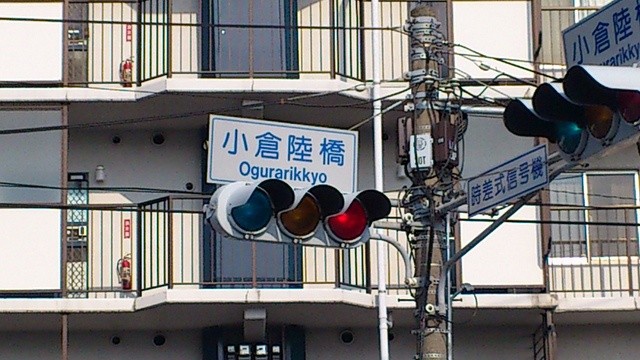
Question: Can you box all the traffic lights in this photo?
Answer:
[503,64,640,159]
[202,176,391,249]
